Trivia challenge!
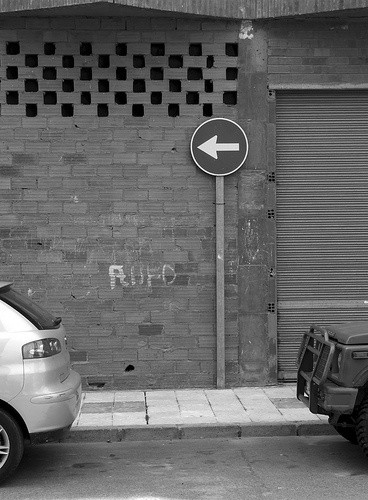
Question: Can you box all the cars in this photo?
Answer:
[0,282,81,483]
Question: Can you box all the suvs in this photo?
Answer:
[296,323,367,456]
